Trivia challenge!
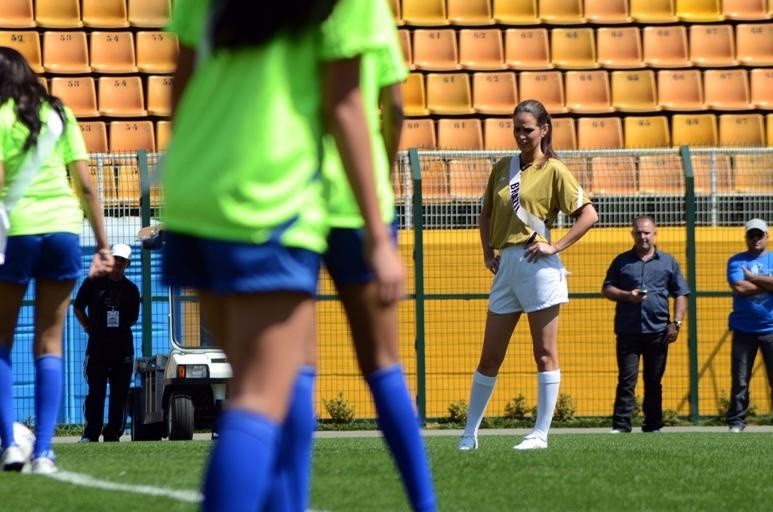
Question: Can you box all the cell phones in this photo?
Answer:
[638,288,648,296]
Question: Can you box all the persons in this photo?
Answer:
[727,218,773,433]
[258,0,440,512]
[73,243,140,444]
[601,215,691,434]
[1,46,116,476]
[456,98,599,451]
[158,0,404,512]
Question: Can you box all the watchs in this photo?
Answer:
[672,320,682,327]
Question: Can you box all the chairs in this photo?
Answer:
[0,1,773,209]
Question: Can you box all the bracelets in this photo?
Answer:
[96,249,113,254]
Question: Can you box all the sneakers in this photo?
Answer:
[31,457,58,475]
[1,445,24,471]
[512,434,548,450]
[456,435,478,450]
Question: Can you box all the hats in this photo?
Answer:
[112,244,131,260]
[744,218,767,233]
